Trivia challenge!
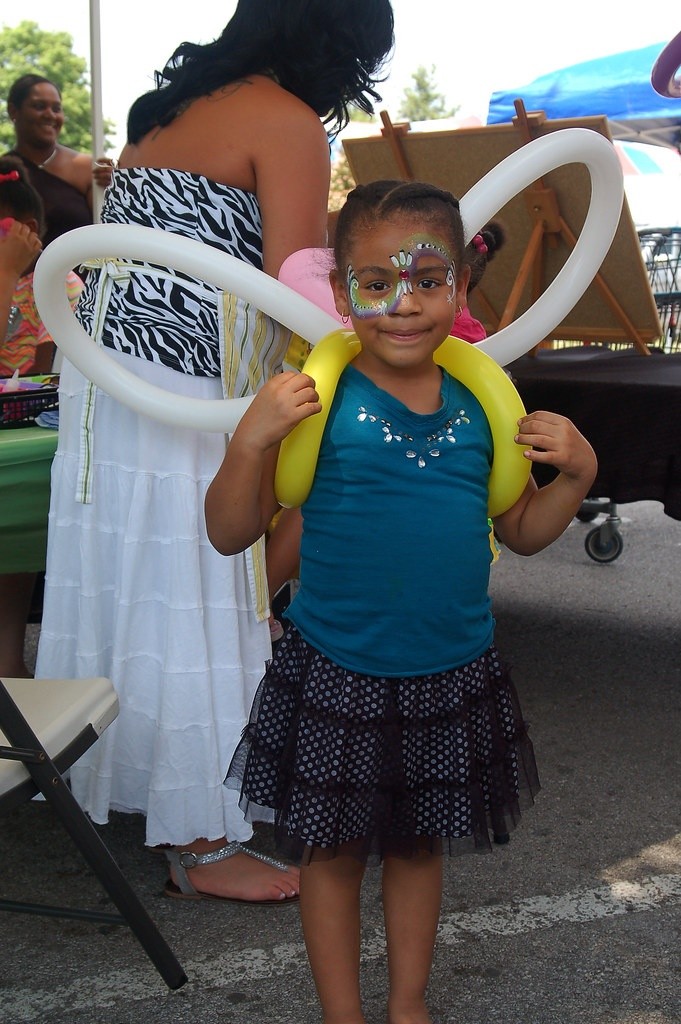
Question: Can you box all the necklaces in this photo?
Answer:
[37,148,57,169]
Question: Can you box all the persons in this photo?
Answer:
[32,1,398,906]
[203,180,600,1024]
[0,74,116,676]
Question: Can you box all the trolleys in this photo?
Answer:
[553,229,679,562]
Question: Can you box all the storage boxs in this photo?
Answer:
[0,372,59,430]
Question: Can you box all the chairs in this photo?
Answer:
[0,675,186,991]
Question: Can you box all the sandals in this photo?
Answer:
[163,841,300,905]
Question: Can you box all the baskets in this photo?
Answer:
[0,373,61,430]
